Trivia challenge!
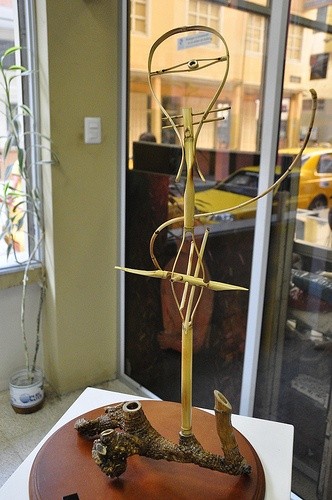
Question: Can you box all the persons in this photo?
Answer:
[299,138,303,148]
[141,133,157,143]
[312,140,318,147]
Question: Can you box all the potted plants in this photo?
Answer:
[0,45,49,414]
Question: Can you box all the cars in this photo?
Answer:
[299,148,332,217]
[170,161,298,228]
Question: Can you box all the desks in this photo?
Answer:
[0,385,295,500]
